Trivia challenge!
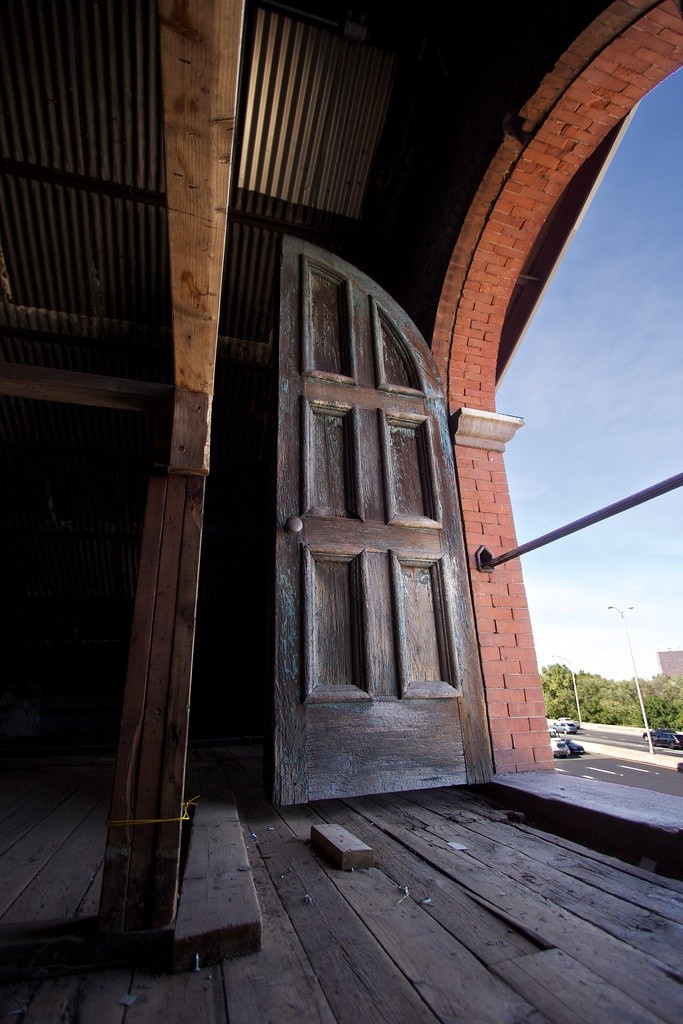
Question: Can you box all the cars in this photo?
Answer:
[552,717,580,734]
[642,729,683,749]
[550,738,584,757]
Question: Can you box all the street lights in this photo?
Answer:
[605,605,655,754]
[551,650,583,729]
